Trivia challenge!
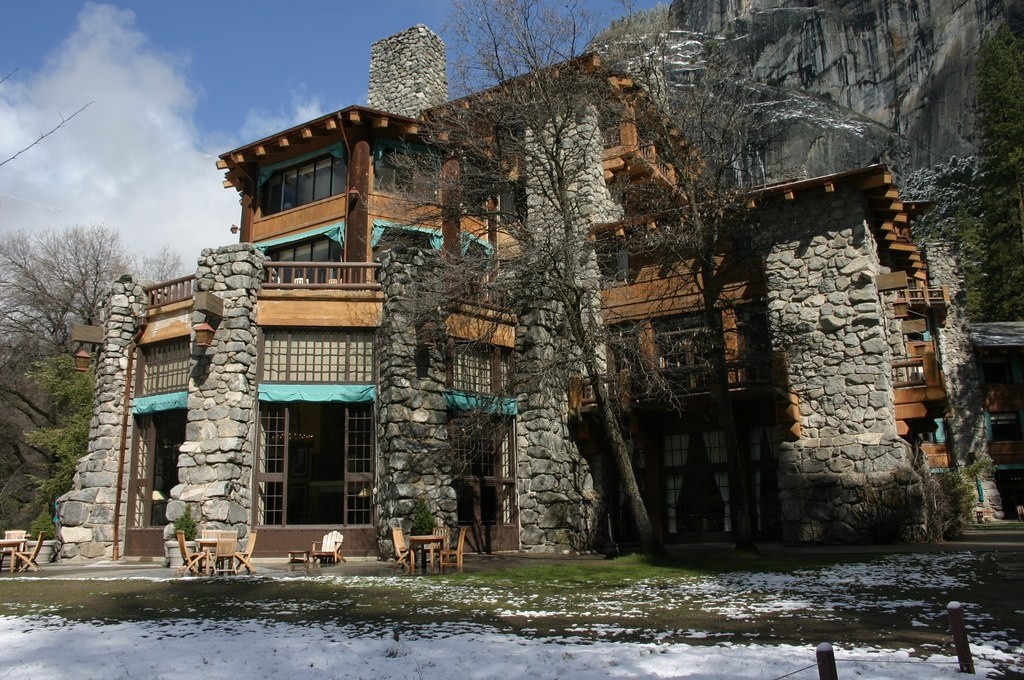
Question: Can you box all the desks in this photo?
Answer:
[407,535,446,575]
[0,539,28,574]
[287,551,311,577]
[195,539,235,575]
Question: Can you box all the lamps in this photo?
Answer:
[230,224,241,234]
[194,320,216,347]
[75,343,91,371]
[350,187,359,198]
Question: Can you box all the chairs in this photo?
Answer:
[176,530,258,576]
[311,531,346,564]
[391,527,467,575]
[0,530,48,571]
